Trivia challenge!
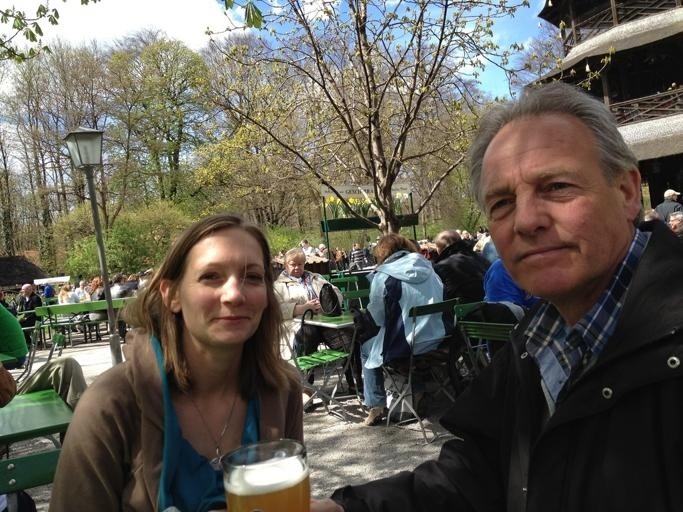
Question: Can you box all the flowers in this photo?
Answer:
[319,192,407,222]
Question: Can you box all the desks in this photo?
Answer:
[1,388,74,458]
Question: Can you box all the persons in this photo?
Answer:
[17,356,89,444]
[645,189,683,238]
[14,283,42,350]
[44,267,154,338]
[311,80,683,511]
[47,215,311,512]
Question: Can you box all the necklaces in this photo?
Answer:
[186,388,238,465]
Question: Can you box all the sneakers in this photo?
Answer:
[365,407,383,426]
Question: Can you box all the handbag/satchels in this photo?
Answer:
[354,312,380,345]
[319,283,341,316]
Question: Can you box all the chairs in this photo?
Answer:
[384,297,460,444]
[1,275,156,347]
[0,448,63,512]
[269,269,528,414]
[279,322,351,423]
[1,318,65,396]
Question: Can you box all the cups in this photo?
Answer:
[669,211,682,237]
[644,208,656,221]
[218,438,311,511]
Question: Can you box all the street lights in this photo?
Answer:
[62,120,124,368]
